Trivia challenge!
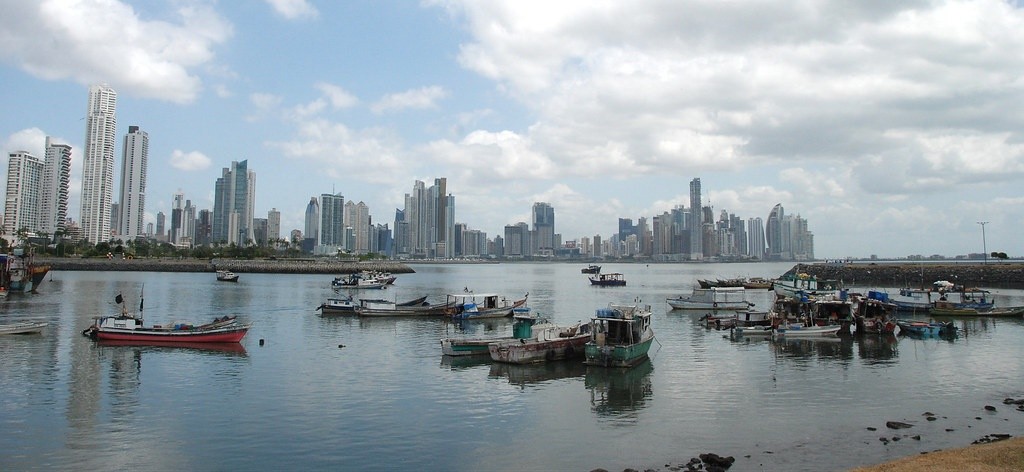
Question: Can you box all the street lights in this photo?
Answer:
[977,221,989,265]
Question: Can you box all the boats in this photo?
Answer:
[80,283,251,342]
[0,245,51,294]
[0,321,49,335]
[216,269,239,283]
[0,287,9,299]
[314,287,529,320]
[588,273,627,286]
[332,269,387,289]
[664,270,1024,340]
[90,338,247,355]
[440,296,656,368]
[581,264,602,274]
[344,270,397,285]
